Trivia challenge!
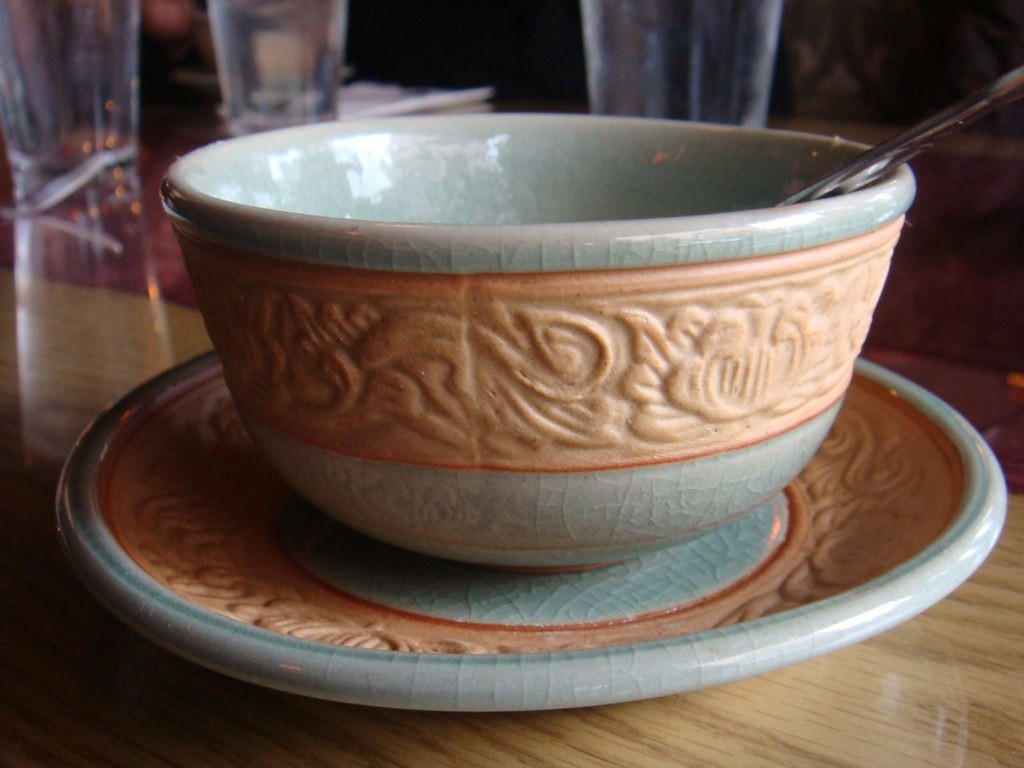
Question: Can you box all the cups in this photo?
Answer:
[580,0,784,128]
[205,0,347,137]
[160,112,918,573]
[0,0,141,206]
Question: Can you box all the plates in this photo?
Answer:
[52,348,1008,711]
[218,83,494,113]
